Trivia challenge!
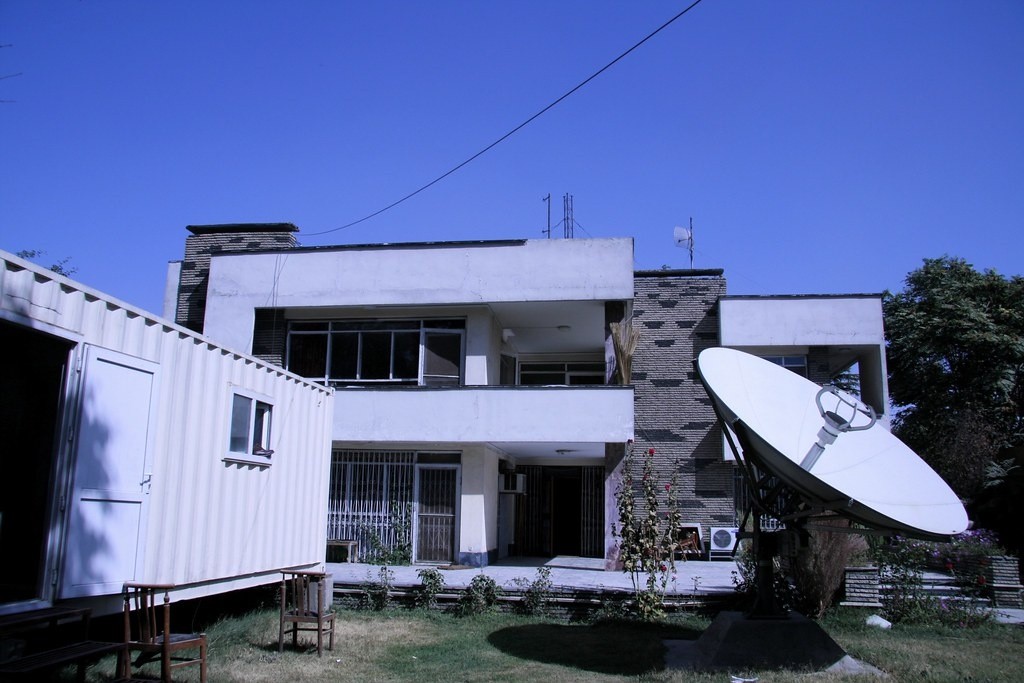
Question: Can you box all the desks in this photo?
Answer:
[327,540,359,563]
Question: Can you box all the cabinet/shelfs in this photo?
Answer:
[671,523,707,553]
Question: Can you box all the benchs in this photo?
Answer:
[0,607,125,683]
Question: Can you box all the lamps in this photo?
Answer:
[502,328,516,342]
[817,364,832,373]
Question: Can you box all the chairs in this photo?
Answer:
[121,582,207,683]
[280,570,335,658]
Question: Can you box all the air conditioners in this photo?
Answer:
[709,527,740,550]
[499,473,527,494]
